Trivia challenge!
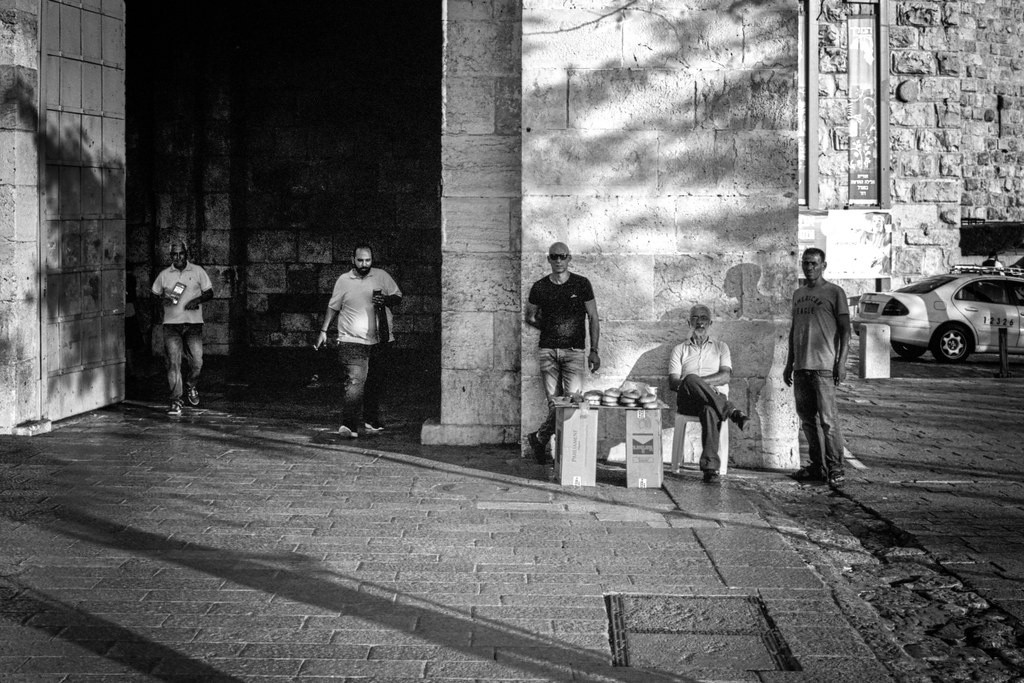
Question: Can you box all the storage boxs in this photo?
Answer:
[554,406,598,487]
[626,409,664,489]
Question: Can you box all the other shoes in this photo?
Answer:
[338,425,358,438]
[528,431,546,465]
[184,380,199,405]
[792,465,826,484]
[167,401,182,415]
[545,448,554,464]
[703,469,720,483]
[829,469,845,488]
[731,409,751,431]
[365,419,385,431]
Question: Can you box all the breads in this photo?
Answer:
[584,388,658,408]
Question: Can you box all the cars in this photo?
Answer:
[852,264,1023,366]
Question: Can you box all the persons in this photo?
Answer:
[668,305,751,483]
[982,248,1003,267]
[151,243,214,415]
[784,248,851,488]
[524,243,600,466]
[316,242,403,440]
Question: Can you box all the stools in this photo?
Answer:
[671,411,701,474]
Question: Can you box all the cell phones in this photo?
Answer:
[373,289,381,297]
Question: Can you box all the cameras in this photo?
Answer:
[168,282,187,304]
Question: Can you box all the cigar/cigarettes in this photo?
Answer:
[313,345,318,350]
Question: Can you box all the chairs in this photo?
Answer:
[978,283,1004,303]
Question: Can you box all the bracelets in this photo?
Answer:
[591,348,598,353]
[320,330,328,332]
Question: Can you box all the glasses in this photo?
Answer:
[549,253,569,260]
